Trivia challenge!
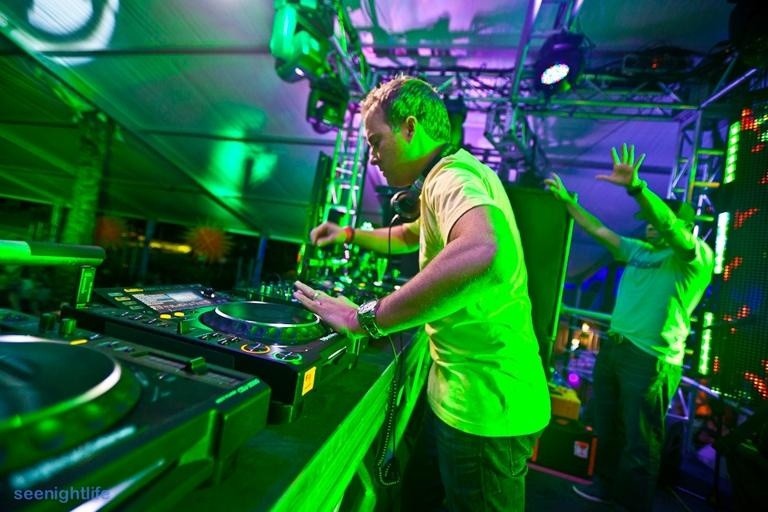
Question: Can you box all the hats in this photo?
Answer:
[634,199,700,225]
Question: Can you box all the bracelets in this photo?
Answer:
[344,225,353,242]
[626,180,646,197]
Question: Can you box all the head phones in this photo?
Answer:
[389,142,460,223]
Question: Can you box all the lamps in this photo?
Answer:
[525,25,585,94]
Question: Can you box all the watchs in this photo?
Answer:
[357,298,383,341]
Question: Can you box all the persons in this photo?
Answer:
[293,74,551,511]
[542,142,716,512]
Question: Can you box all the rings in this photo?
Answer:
[312,292,319,301]
